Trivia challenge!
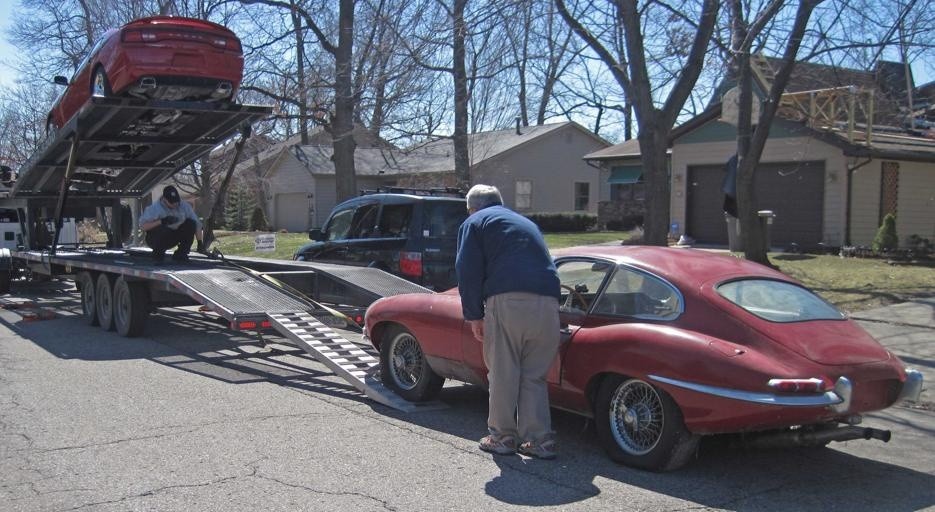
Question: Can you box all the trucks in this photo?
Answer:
[1,95,451,416]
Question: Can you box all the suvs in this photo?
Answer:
[293,185,470,292]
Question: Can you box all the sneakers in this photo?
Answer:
[151,252,165,265]
[172,250,192,265]
[517,439,557,460]
[478,435,516,455]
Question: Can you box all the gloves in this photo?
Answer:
[197,240,205,253]
[161,216,179,224]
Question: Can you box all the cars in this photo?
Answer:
[45,14,244,143]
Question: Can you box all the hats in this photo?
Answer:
[163,186,180,204]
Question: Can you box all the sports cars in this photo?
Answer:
[362,244,922,473]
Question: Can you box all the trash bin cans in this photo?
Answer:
[724,210,776,252]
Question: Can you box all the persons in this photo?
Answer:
[455,183,560,460]
[139,186,205,266]
